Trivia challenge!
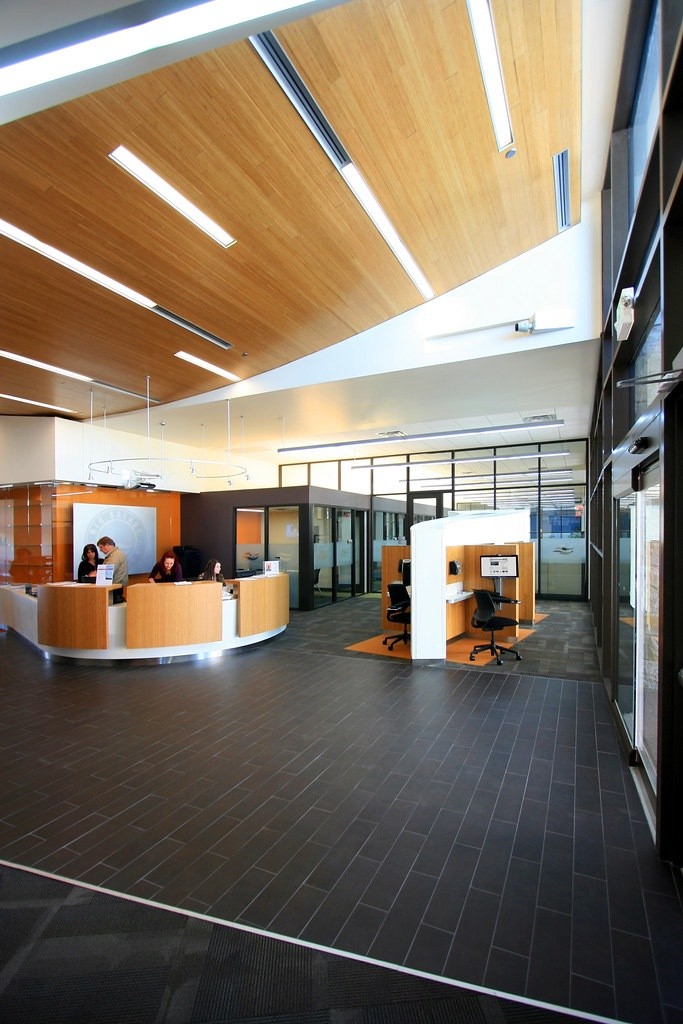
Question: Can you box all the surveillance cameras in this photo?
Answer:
[515,321,532,332]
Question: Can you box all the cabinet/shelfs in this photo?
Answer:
[1,482,55,583]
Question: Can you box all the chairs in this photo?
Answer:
[313,569,324,598]
[469,589,522,664]
[382,583,411,650]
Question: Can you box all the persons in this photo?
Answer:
[148,552,184,582]
[195,560,226,587]
[78,537,129,605]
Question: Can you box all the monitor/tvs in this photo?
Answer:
[479,555,519,578]
[80,576,97,584]
[236,571,256,578]
